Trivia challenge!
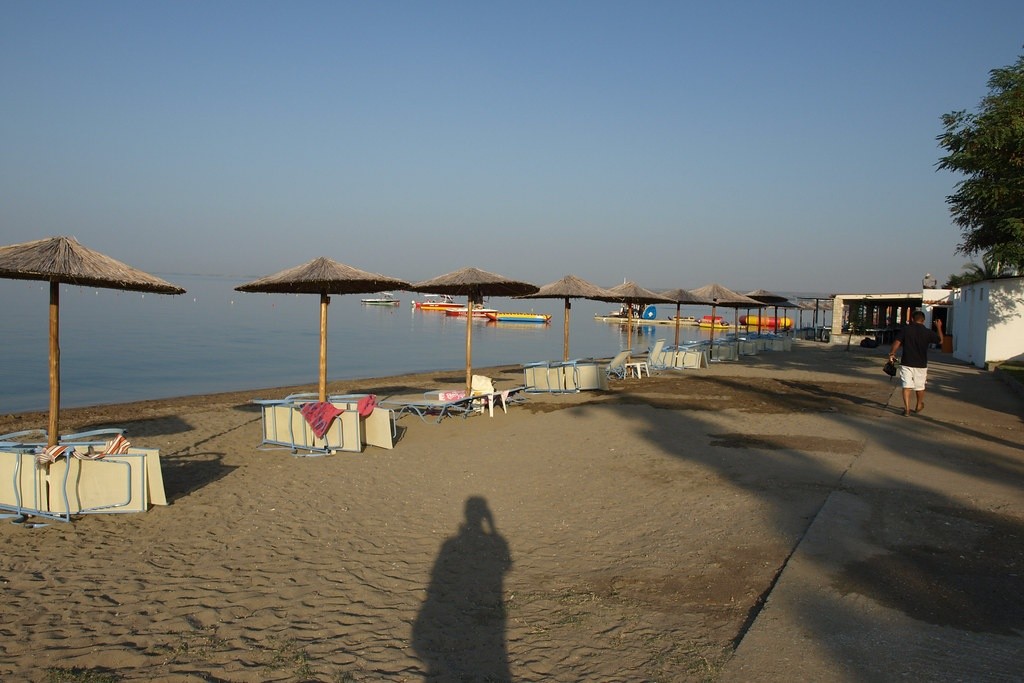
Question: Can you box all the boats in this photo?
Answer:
[361,292,401,305]
[739,315,793,327]
[698,320,732,329]
[445,303,498,316]
[415,293,466,311]
[483,311,552,322]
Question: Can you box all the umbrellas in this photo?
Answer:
[779,301,833,328]
[0,237,187,448]
[588,282,678,362]
[691,284,753,359]
[411,266,540,396]
[744,289,788,334]
[643,287,716,353]
[511,275,623,363]
[234,257,409,403]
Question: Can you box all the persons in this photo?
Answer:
[889,311,943,416]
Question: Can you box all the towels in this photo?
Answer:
[298,393,382,438]
[438,389,488,405]
[34,434,133,470]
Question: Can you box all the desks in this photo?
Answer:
[623,361,650,380]
[865,328,894,345]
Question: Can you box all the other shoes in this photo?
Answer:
[915,402,924,412]
[904,410,910,417]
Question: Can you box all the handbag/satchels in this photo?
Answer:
[883,360,896,376]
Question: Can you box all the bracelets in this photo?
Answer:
[889,353,894,355]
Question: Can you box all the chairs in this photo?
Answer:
[0,428,170,529]
[646,331,792,371]
[605,350,633,381]
[253,358,601,459]
